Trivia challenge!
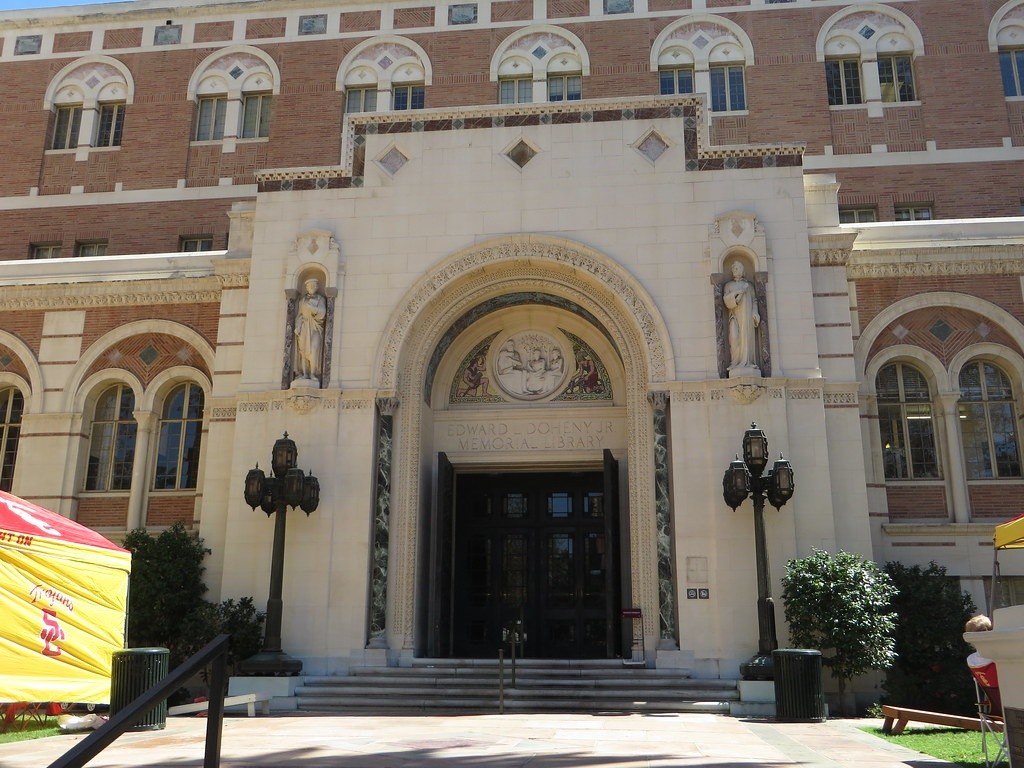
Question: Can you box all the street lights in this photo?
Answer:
[722,421,795,680]
[238,430,321,675]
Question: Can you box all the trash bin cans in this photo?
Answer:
[110,647,172,733]
[770,646,827,722]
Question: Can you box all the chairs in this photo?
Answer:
[971,661,1010,768]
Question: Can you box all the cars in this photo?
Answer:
[919,653,983,717]
[126,447,188,487]
[964,387,989,400]
[886,435,936,476]
[984,438,1019,471]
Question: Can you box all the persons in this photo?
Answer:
[528,350,546,390]
[498,341,528,394]
[539,349,562,394]
[964,616,998,684]
[295,279,327,380]
[724,262,760,369]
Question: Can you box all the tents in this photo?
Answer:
[988,513,1024,617]
[0,490,134,732]
